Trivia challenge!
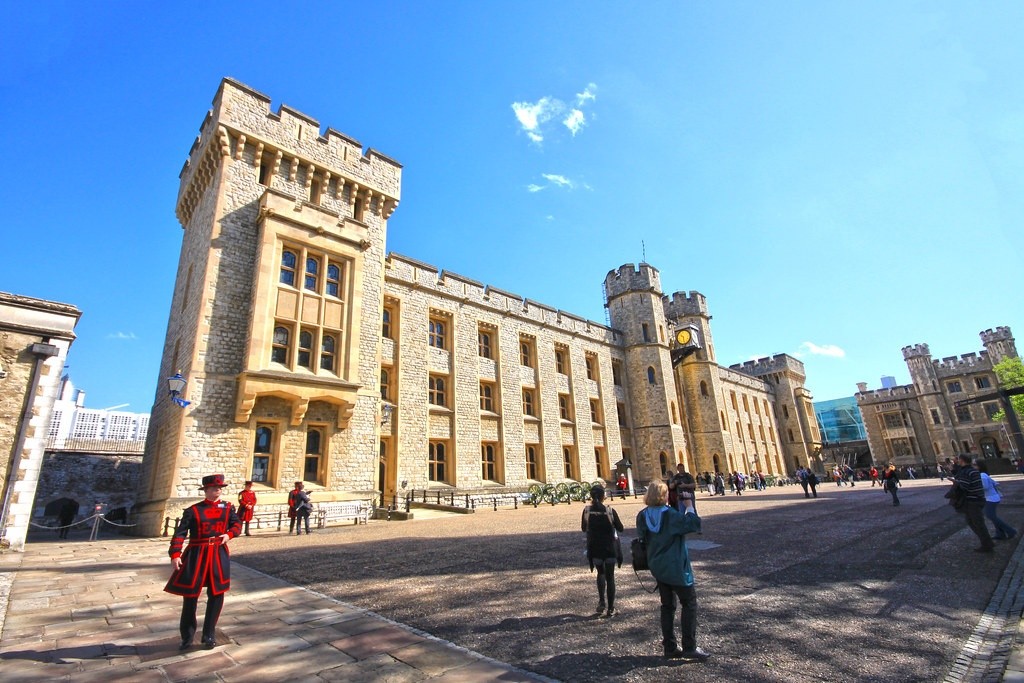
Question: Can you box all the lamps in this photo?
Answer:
[165,369,193,409]
[381,403,392,423]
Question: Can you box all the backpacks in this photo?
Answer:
[800,471,807,480]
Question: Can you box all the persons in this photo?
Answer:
[616,473,626,499]
[237,481,257,537]
[287,482,303,534]
[636,479,711,659]
[665,453,1017,552]
[162,473,242,650]
[581,486,624,616]
[294,484,314,535]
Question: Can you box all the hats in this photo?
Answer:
[197,474,228,490]
[243,481,254,486]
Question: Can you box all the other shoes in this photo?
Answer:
[974,547,994,551]
[682,648,710,658]
[665,646,683,655]
[246,534,251,536]
[1009,531,1018,539]
[991,535,1007,539]
[596,605,604,613]
[608,607,616,615]
[207,637,215,648]
[178,639,192,649]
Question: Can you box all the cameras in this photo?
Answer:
[679,493,694,501]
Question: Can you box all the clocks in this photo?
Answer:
[675,329,692,346]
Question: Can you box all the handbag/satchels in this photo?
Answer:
[631,538,650,570]
